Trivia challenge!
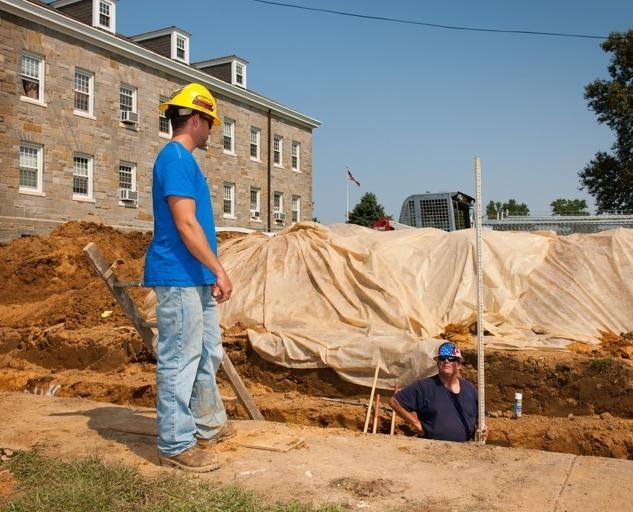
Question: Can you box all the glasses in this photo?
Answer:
[200,114,214,129]
[437,357,460,362]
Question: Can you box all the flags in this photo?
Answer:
[346,169,360,188]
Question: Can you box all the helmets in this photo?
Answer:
[157,81,222,126]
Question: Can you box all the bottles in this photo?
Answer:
[514,392,522,418]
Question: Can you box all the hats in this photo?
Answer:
[432,342,463,361]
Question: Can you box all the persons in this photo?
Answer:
[387,342,488,443]
[143,82,240,475]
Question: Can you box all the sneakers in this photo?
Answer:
[157,445,223,473]
[196,420,239,449]
